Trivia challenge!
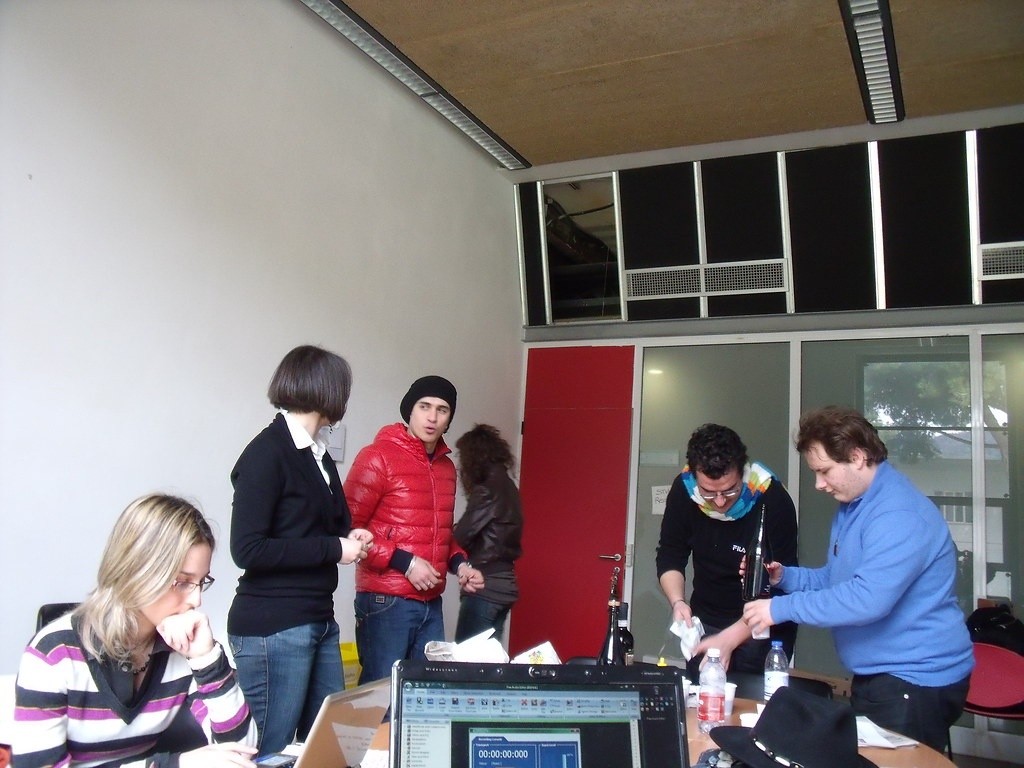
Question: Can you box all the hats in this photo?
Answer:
[400,376,457,434]
[710,686,880,768]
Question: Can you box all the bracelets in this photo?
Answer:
[671,599,686,608]
[405,555,417,578]
[457,562,473,568]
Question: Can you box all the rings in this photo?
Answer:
[428,581,430,587]
[356,557,361,564]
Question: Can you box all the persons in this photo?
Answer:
[450,425,523,646]
[227,345,375,758]
[341,376,485,688]
[7,493,259,768]
[654,423,799,677]
[741,404,976,754]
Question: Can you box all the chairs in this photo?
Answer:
[945,641,1024,768]
[35,603,208,759]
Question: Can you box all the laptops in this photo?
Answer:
[390,659,690,768]
[252,674,391,768]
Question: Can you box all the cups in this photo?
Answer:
[723,682,738,715]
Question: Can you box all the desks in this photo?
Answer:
[359,697,959,768]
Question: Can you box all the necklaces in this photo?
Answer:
[129,652,150,674]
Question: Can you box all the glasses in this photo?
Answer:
[697,475,740,500]
[151,564,214,596]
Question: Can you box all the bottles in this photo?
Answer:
[697,648,727,734]
[763,641,789,703]
[616,602,635,667]
[741,503,774,602]
